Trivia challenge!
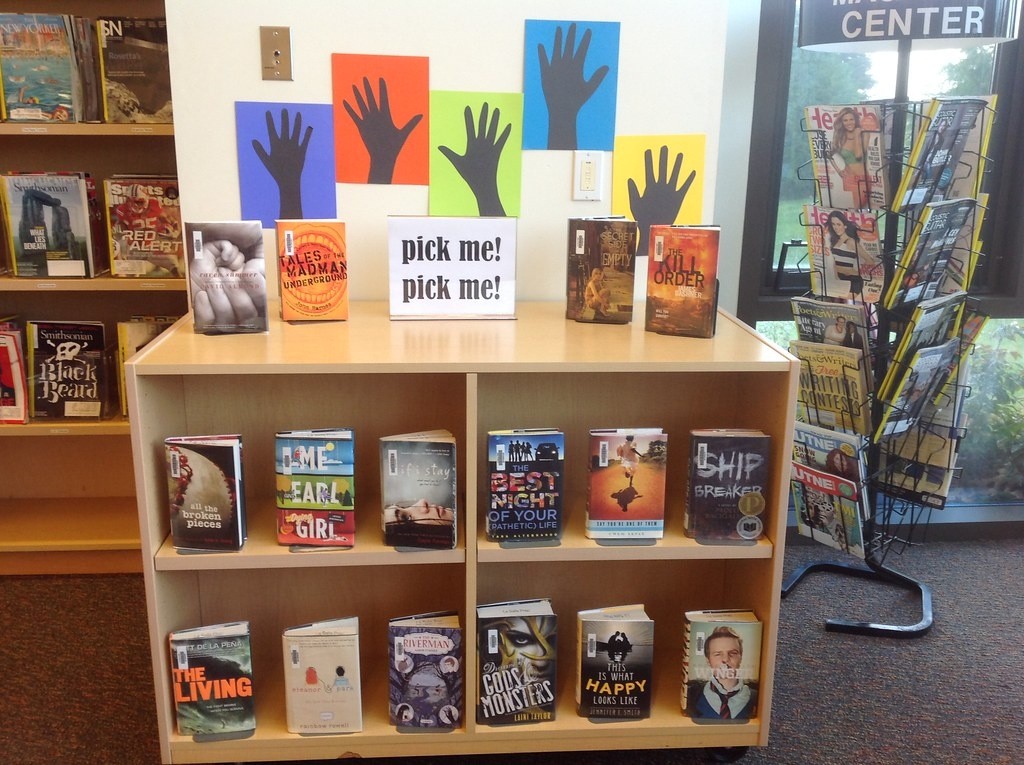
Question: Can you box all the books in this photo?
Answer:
[158,426,775,723]
[790,96,987,560]
[0,13,189,424]
[275,218,348,321]
[644,224,721,338]
[181,217,270,335]
[567,215,638,325]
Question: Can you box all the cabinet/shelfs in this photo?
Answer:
[0,0,192,575]
[123,300,801,765]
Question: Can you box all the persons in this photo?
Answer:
[383,500,456,550]
[823,212,875,305]
[827,109,878,209]
[616,436,646,488]
[822,315,846,346]
[185,221,269,333]
[840,321,863,349]
[584,268,610,317]
[490,617,557,714]
[686,626,760,720]
[824,450,857,483]
[905,118,947,191]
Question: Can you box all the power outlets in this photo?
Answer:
[572,151,602,200]
